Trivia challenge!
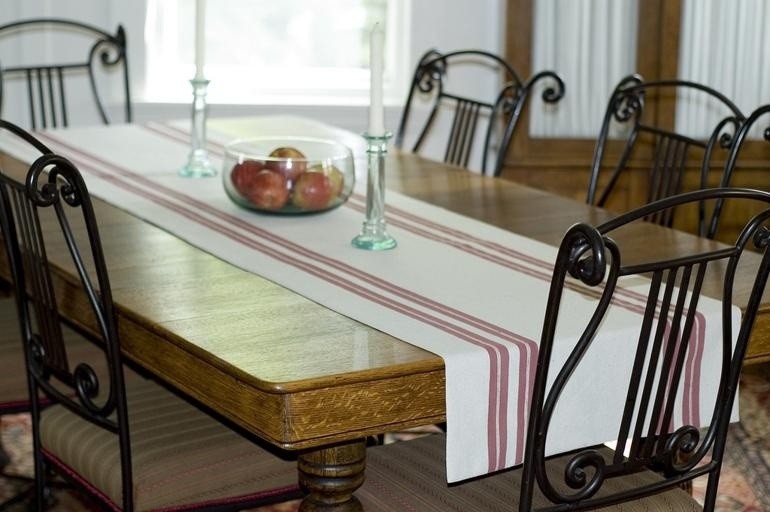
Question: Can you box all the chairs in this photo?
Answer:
[0,19,132,127]
[0,395,82,512]
[0,119,298,512]
[396,50,564,178]
[585,73,770,237]
[353,187,770,512]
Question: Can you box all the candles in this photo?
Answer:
[370,24,385,136]
[194,0,205,81]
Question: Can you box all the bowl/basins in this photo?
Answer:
[222,135,357,216]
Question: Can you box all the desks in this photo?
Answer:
[0,115,770,512]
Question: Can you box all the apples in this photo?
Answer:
[291,172,333,210]
[265,148,305,181]
[307,165,345,200]
[231,158,262,196]
[247,170,288,208]
